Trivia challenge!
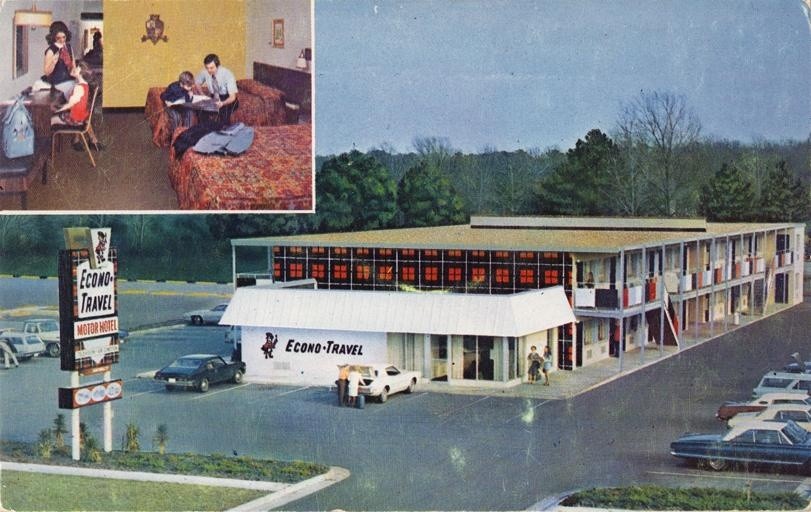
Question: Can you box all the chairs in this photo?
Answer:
[51,87,99,169]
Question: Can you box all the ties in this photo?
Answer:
[212,77,220,101]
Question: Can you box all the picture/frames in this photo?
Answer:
[272,19,285,48]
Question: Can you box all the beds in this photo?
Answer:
[144,79,288,148]
[168,122,313,210]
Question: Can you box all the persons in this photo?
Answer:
[346,366,366,407]
[337,364,348,406]
[85,32,103,57]
[527,346,541,385]
[49,61,99,130]
[159,71,195,144]
[44,21,103,152]
[612,319,620,357]
[0,330,19,369]
[195,54,238,127]
[585,272,594,288]
[542,346,552,386]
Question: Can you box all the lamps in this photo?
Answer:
[296,48,310,68]
[14,0,53,30]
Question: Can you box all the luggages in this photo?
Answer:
[357,395,365,409]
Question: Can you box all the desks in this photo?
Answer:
[0,137,50,211]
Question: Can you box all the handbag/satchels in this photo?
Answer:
[1,97,35,158]
